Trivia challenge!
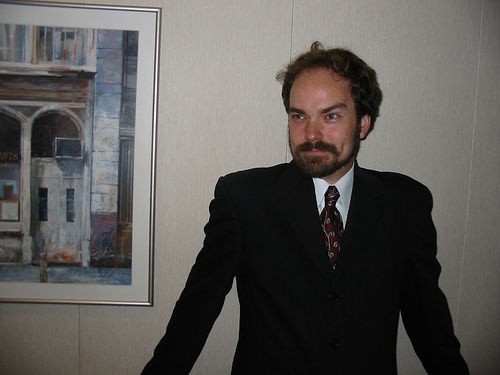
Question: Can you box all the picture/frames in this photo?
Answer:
[1,0,163,306]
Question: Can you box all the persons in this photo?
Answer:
[138,40,471,375]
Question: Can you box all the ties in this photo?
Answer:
[320,185,344,274]
